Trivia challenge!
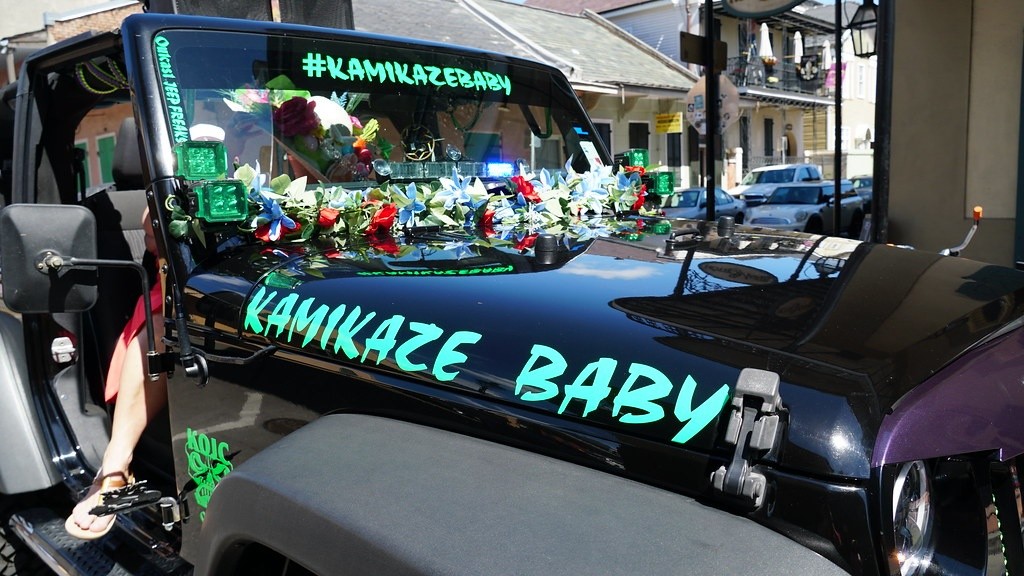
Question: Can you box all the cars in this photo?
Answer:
[724,164,824,206]
[743,178,865,240]
[848,174,874,212]
[650,188,745,226]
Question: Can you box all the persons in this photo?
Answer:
[778,123,798,164]
[65,94,360,542]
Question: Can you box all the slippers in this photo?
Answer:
[65,470,137,539]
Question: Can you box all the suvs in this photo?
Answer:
[3,11,1021,576]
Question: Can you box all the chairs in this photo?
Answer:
[83,113,169,465]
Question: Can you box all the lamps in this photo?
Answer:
[809,256,848,280]
[841,0,880,60]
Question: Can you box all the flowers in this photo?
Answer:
[220,73,396,179]
[231,159,655,246]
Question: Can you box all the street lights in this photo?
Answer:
[835,0,881,239]
